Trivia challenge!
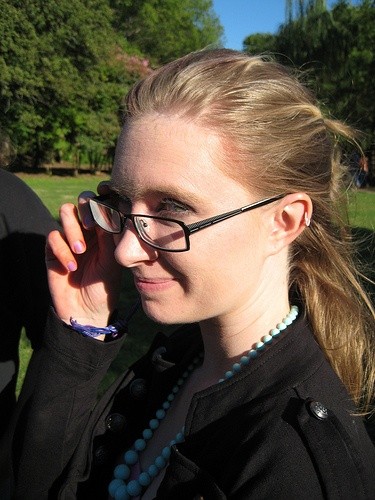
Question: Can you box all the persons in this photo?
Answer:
[2,160,83,423]
[3,45,375,500]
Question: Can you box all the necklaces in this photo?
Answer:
[108,305,300,500]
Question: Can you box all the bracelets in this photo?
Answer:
[65,315,125,339]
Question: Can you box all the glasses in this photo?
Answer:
[85,187,281,257]
